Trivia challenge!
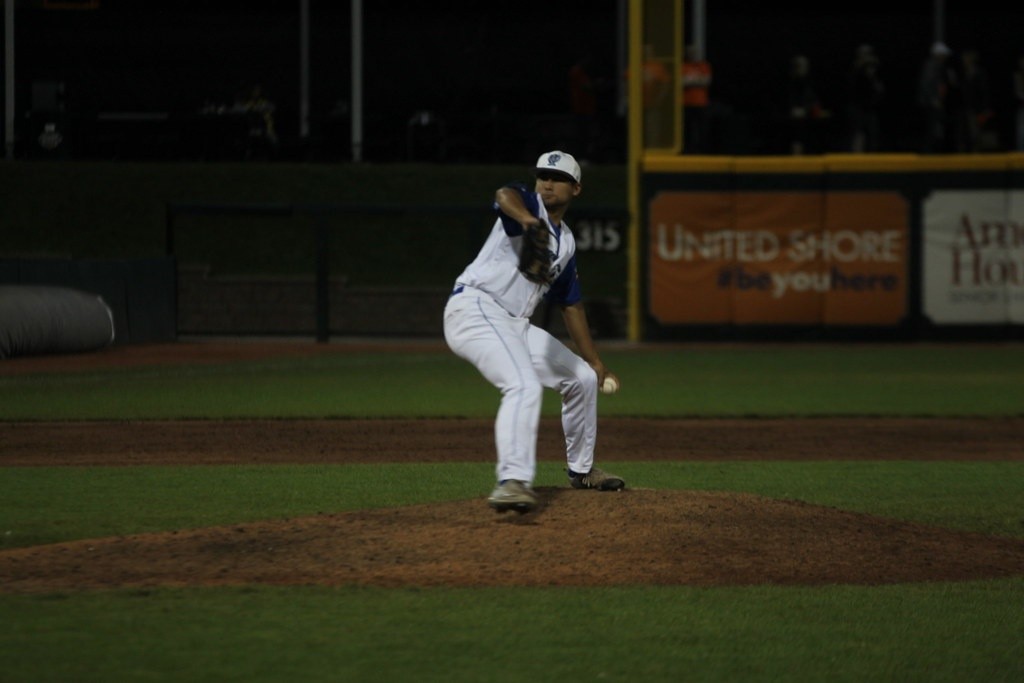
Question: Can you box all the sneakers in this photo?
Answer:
[562,467,625,490]
[486,479,539,509]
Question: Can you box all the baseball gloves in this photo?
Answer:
[515,216,557,294]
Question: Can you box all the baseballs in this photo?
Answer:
[598,376,618,395]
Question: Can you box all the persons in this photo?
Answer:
[443,150,626,511]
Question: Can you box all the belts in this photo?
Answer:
[451,286,463,296]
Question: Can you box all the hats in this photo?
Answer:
[532,150,582,186]
